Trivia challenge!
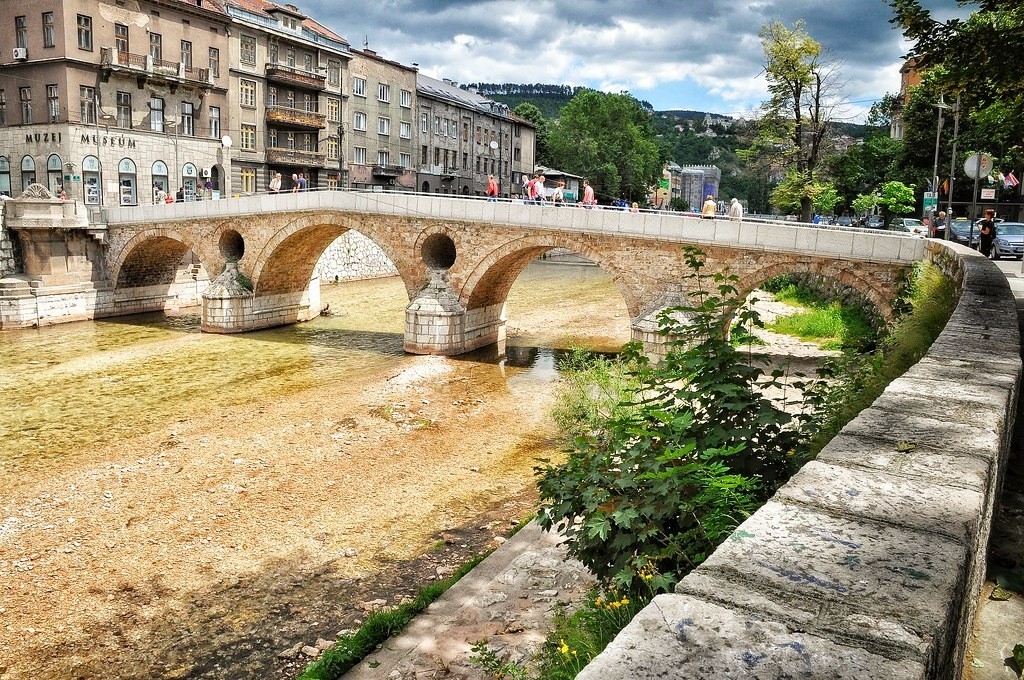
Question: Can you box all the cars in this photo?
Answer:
[974,218,1005,223]
[836,214,885,229]
[945,217,981,250]
[979,222,1024,261]
[888,217,934,239]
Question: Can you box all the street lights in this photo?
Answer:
[313,58,346,191]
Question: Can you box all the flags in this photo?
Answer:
[988,171,1019,187]
[926,179,948,195]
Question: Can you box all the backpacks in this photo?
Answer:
[524,182,528,196]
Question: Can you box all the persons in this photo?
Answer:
[60,190,67,200]
[814,213,821,224]
[155,173,308,206]
[485,175,743,222]
[934,209,995,257]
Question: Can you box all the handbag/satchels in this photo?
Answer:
[488,181,494,196]
[551,188,560,201]
[992,227,997,239]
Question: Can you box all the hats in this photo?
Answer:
[276,173,282,176]
[730,198,738,203]
[707,195,713,200]
